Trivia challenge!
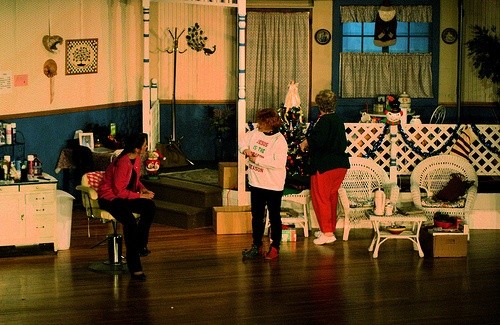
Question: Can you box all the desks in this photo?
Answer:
[263,190,310,237]
[54,147,115,193]
[363,210,427,259]
[361,111,414,117]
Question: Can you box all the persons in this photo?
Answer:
[284,80,301,108]
[238,108,288,260]
[146,150,166,176]
[300,90,351,245]
[98,135,155,282]
[385,100,403,122]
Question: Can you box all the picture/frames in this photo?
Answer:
[441,28,457,44]
[79,132,94,149]
[314,29,330,45]
[65,38,99,75]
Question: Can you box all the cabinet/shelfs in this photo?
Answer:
[0,131,58,256]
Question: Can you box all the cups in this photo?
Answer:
[386,206,392,216]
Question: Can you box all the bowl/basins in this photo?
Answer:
[387,227,406,235]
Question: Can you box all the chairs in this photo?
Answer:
[410,155,478,241]
[338,157,399,241]
[429,105,446,124]
[76,171,140,275]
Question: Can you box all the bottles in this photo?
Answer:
[0,155,42,180]
[2,122,16,145]
[374,187,386,216]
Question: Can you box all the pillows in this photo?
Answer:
[431,172,475,204]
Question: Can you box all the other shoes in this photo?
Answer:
[140,246,152,257]
[263,247,279,259]
[130,270,147,278]
[242,244,264,259]
[313,231,336,244]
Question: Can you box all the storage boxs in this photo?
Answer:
[427,231,467,258]
[281,230,297,242]
[212,206,252,235]
[218,162,238,189]
